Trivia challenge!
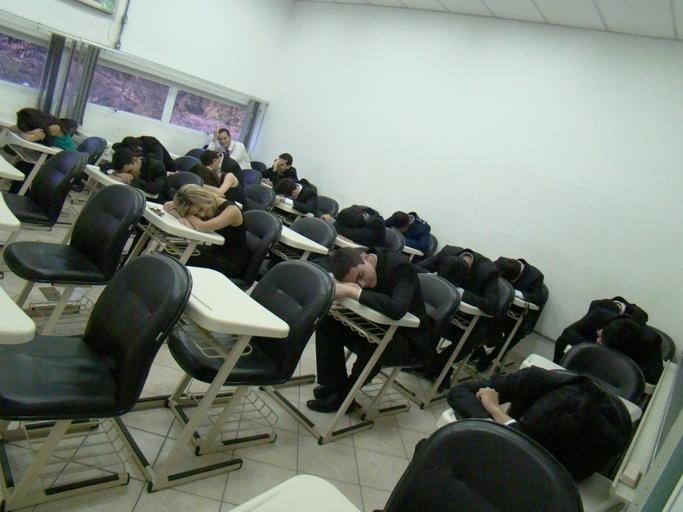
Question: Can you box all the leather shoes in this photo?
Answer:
[469,345,487,360]
[437,363,454,393]
[306,390,345,413]
[312,381,350,400]
[477,354,491,373]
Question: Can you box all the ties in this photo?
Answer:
[226,149,230,158]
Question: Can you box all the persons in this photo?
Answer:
[553,305,663,385]
[9,107,430,278]
[471,254,544,373]
[416,245,500,391]
[307,248,432,412]
[448,365,623,483]
[588,296,648,326]
